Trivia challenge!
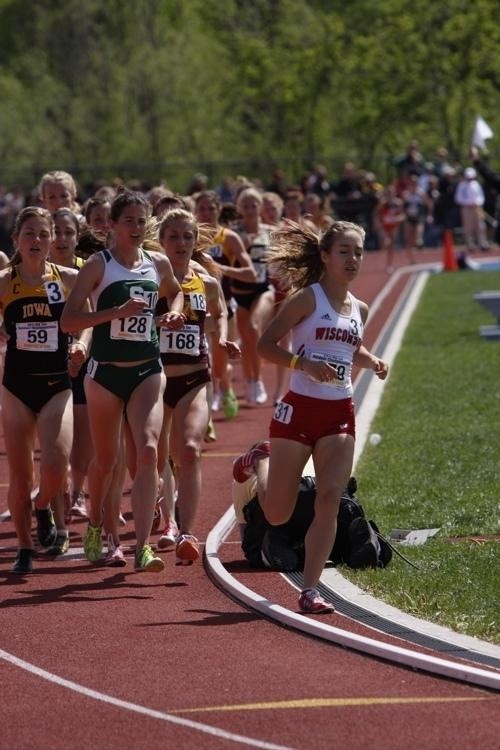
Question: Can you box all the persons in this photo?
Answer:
[0,166,329,576]
[309,137,499,267]
[233,213,388,614]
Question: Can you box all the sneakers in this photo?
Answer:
[12,547,38,574]
[82,524,102,562]
[174,534,200,564]
[203,376,283,444]
[65,482,177,533]
[298,588,335,615]
[158,524,180,549]
[232,440,270,484]
[34,494,57,548]
[104,545,127,567]
[133,544,165,574]
[48,535,70,557]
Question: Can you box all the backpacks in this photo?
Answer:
[240,475,402,573]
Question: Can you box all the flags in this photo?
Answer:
[469,114,496,153]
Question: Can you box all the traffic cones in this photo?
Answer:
[438,229,460,273]
